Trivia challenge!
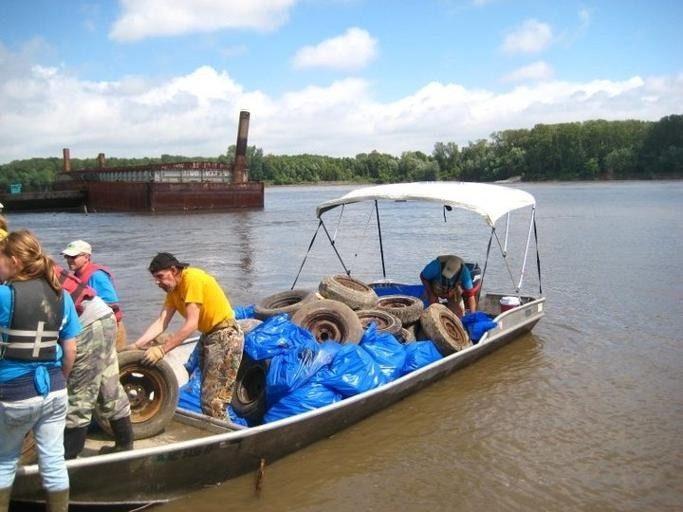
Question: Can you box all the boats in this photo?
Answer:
[10,180,546,505]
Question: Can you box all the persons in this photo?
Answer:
[0,210,11,242]
[418,255,477,321]
[59,238,122,327]
[40,258,137,459]
[127,250,246,424]
[0,228,83,512]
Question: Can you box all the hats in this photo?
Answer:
[442,257,462,280]
[148,252,190,274]
[59,239,92,257]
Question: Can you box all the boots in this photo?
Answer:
[99,415,134,455]
